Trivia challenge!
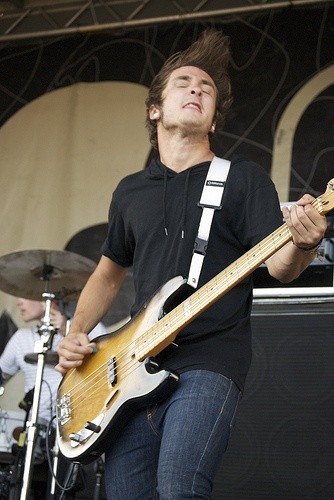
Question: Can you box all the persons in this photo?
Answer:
[55,27,327,500]
[0,297,107,500]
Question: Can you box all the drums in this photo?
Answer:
[1,408,54,465]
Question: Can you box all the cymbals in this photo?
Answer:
[0,249,98,302]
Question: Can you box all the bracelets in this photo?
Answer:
[299,237,324,254]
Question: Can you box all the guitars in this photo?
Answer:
[55,178,334,464]
